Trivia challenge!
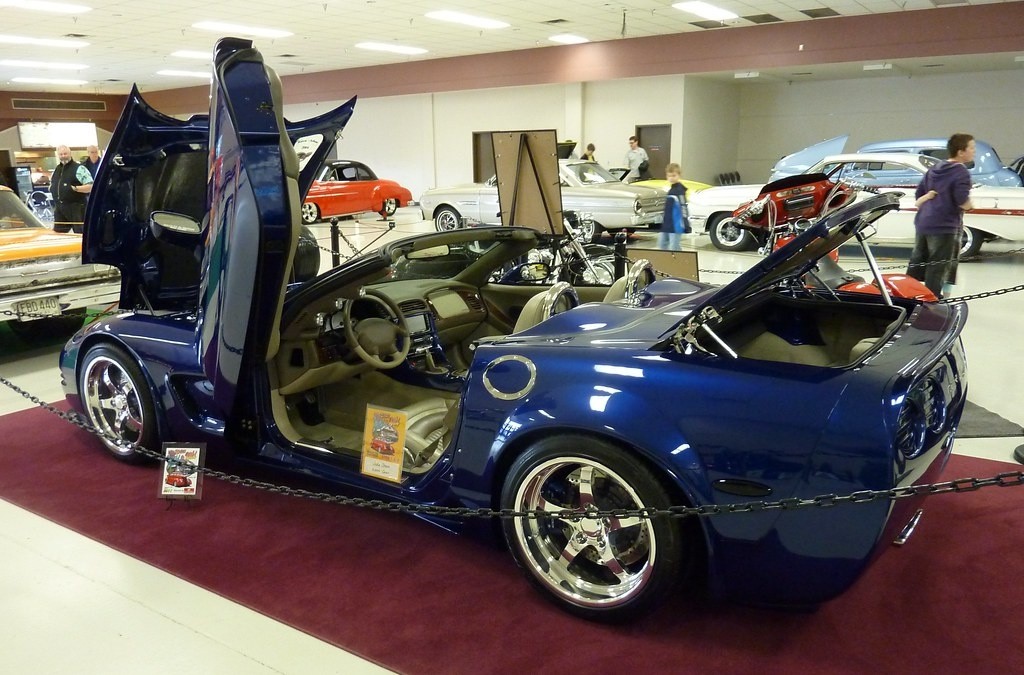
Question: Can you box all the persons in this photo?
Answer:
[580,143,596,174]
[907,133,975,297]
[624,135,649,184]
[48,145,93,235]
[83,146,103,180]
[657,163,692,250]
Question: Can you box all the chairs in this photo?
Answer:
[399,291,567,471]
[601,274,646,302]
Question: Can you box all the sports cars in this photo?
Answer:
[58,36,969,625]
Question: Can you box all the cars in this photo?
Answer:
[688,154,1024,258]
[767,133,1023,190]
[300,159,416,225]
[0,182,124,348]
[419,159,667,244]
